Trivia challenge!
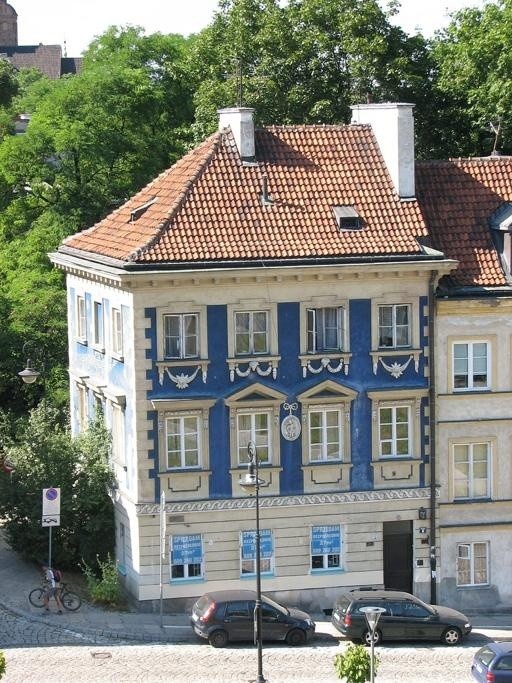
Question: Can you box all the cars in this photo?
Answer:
[470,642,512,683]
[190,589,314,646]
[331,588,472,646]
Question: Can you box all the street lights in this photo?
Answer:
[240,441,265,682]
[359,606,386,682]
[18,358,49,420]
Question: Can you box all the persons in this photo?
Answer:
[42,564,63,615]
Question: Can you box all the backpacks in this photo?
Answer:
[52,569,63,582]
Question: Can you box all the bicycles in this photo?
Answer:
[28,579,82,611]
